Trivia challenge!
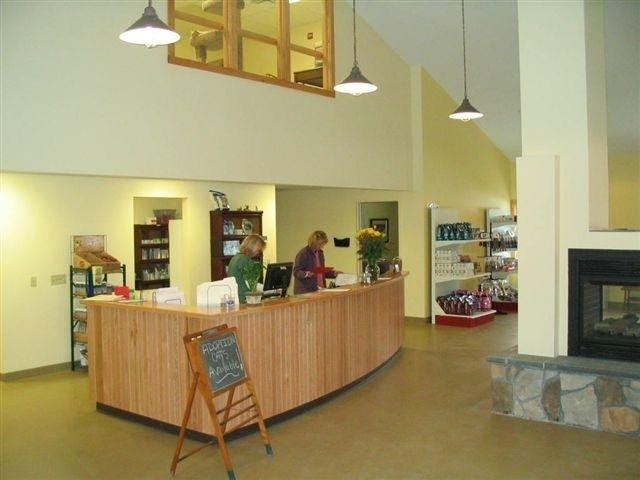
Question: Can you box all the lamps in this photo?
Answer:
[118,0,180,48]
[333,0,378,97]
[448,0,484,122]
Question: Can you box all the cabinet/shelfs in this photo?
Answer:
[430,207,497,328]
[134,225,169,289]
[210,210,262,285]
[485,208,519,314]
[69,265,126,371]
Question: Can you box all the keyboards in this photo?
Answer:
[261,292,279,299]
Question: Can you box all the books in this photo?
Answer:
[141,248,169,260]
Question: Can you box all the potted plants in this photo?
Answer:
[238,260,266,304]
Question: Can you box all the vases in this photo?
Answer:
[364,263,379,285]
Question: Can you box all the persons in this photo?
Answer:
[228,234,266,305]
[293,231,344,295]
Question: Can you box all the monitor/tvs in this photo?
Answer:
[263,262,293,297]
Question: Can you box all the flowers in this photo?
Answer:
[356,227,388,263]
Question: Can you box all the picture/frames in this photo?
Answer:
[371,218,389,244]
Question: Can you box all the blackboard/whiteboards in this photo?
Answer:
[186,327,251,399]
